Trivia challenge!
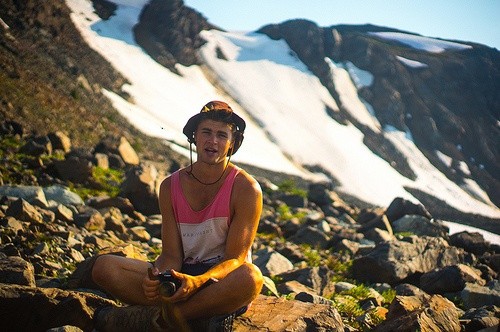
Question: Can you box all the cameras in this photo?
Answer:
[148,269,182,297]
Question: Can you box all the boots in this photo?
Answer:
[94,304,168,332]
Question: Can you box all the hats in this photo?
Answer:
[183,101,246,156]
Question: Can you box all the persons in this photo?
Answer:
[92,102,264,331]
